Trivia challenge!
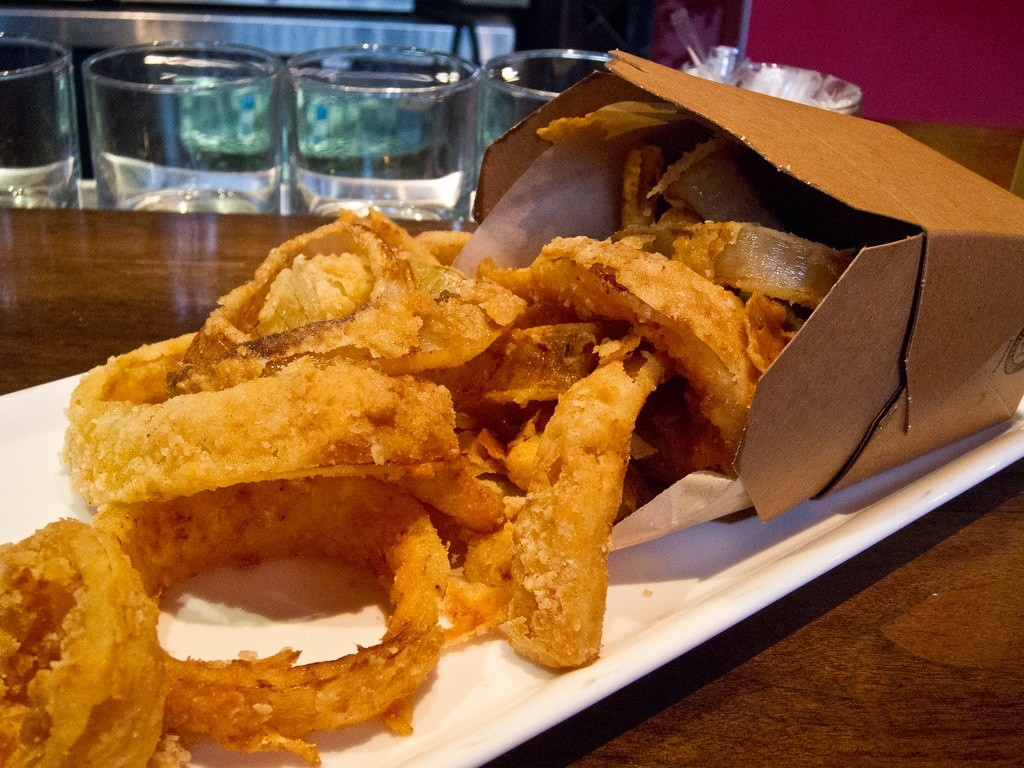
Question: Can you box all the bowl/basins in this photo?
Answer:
[682,62,862,115]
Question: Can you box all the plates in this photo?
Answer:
[0,350,1024,767]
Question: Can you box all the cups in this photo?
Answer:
[0,34,81,209]
[82,41,288,214]
[288,45,482,222]
[479,49,616,166]
